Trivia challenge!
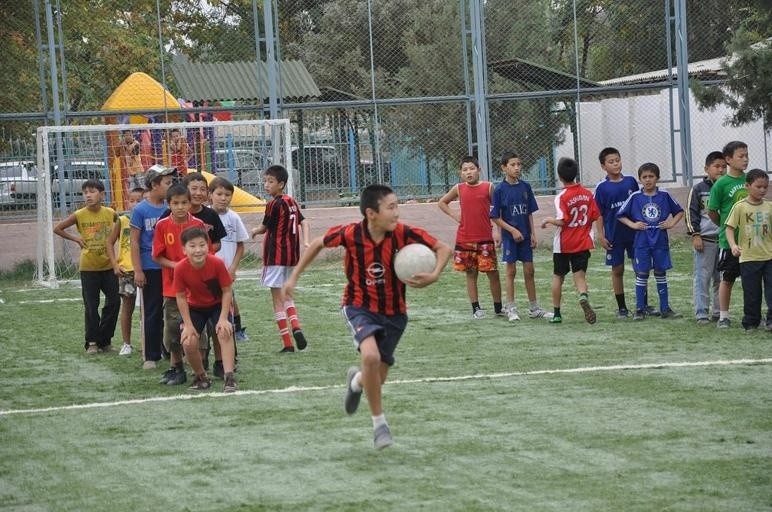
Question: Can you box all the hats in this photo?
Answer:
[143,164,177,188]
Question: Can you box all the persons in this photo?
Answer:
[592,148,661,316]
[250,165,307,353]
[52,179,120,352]
[685,152,728,324]
[541,158,600,324]
[707,141,750,328]
[438,157,510,321]
[281,185,453,449]
[105,164,249,392]
[117,130,193,193]
[489,153,555,322]
[615,163,684,321]
[723,169,772,332]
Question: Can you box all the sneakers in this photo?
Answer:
[473,304,562,323]
[280,347,294,354]
[143,360,156,369]
[697,317,771,332]
[618,305,683,321]
[87,342,100,354]
[344,365,363,414]
[371,424,392,450]
[578,296,596,324]
[236,327,251,341]
[119,342,134,356]
[159,359,238,394]
[291,326,306,351]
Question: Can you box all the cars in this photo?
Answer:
[0,161,39,205]
[280,145,389,184]
[53,161,105,198]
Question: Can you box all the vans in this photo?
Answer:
[215,149,299,197]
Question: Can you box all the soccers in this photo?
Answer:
[393,243,437,284]
